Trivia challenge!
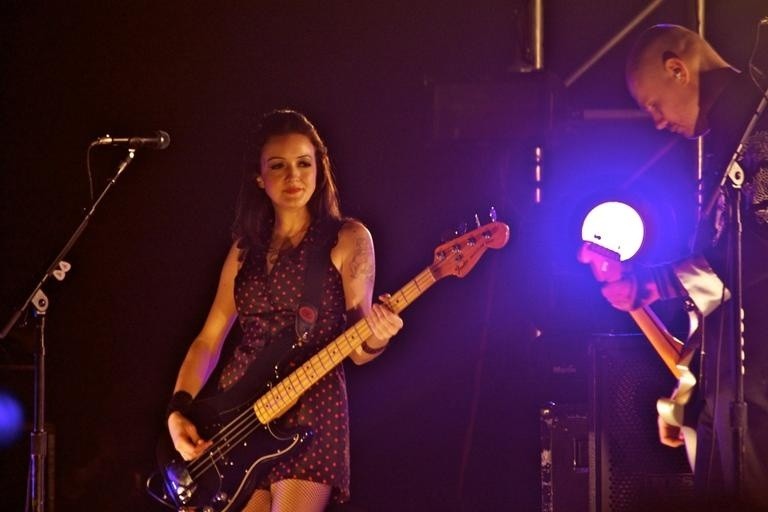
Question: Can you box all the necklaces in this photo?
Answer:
[268,220,315,264]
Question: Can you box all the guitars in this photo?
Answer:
[155,205,512,510]
[577,241,700,472]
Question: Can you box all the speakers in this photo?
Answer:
[539,401,588,512]
[588,331,696,512]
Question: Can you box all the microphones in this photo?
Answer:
[96,129,171,150]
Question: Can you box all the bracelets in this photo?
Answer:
[362,340,390,355]
[165,390,193,422]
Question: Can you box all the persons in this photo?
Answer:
[164,108,404,512]
[600,23,768,512]
